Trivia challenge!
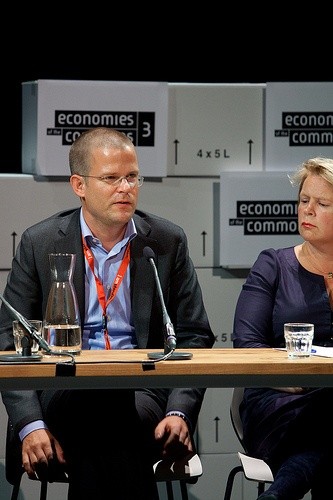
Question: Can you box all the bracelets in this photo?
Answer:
[167,412,191,430]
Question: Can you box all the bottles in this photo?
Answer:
[44,254,82,356]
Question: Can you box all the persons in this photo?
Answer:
[233,156,333,500]
[0,127,216,500]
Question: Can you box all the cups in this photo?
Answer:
[13,320,42,356]
[285,323,313,358]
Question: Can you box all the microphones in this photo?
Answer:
[144,247,194,359]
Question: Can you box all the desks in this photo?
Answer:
[0,348,333,392]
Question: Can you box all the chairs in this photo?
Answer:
[223,387,274,500]
[10,462,199,500]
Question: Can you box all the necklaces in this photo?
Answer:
[301,245,333,279]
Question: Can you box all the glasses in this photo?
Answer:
[72,168,147,189]
